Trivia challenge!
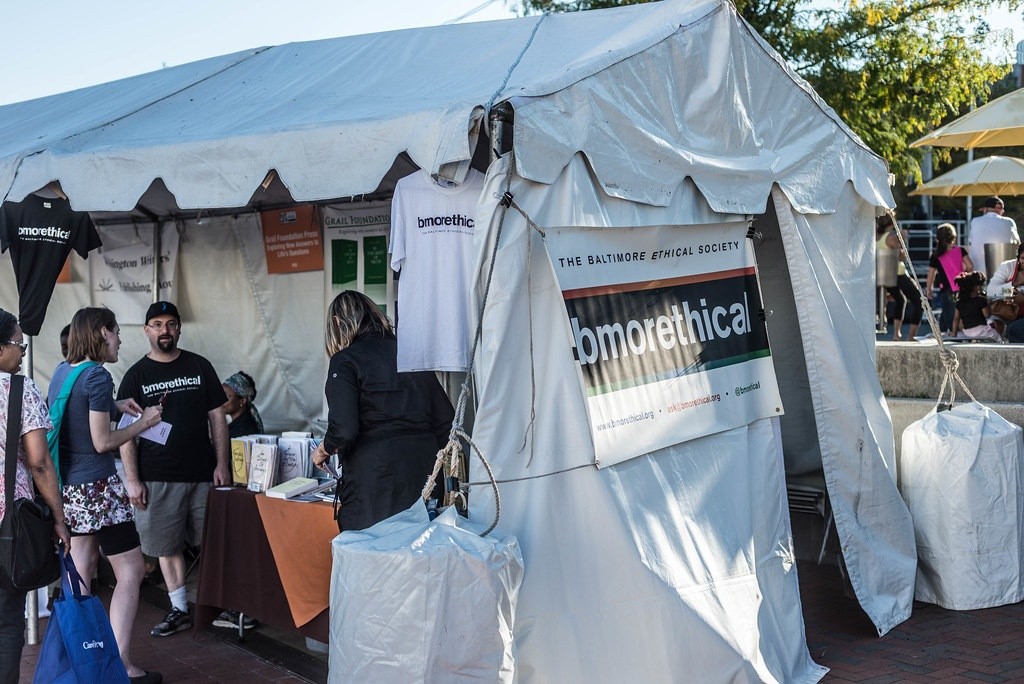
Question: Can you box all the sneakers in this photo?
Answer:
[211,609,259,629]
[150,607,191,637]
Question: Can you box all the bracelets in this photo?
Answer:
[317,446,325,457]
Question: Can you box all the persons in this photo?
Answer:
[311,289,456,532]
[986,241,1024,343]
[926,222,976,344]
[207,370,265,483]
[950,269,1005,343]
[46,306,163,684]
[0,309,70,684]
[879,209,923,341]
[966,196,1022,277]
[117,301,258,636]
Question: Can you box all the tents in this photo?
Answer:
[0,0,920,684]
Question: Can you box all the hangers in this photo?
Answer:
[47,181,66,200]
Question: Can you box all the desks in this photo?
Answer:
[189,480,339,649]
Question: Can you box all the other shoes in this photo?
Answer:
[128,669,163,684]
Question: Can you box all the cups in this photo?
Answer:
[1001,285,1014,301]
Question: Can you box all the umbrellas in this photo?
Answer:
[908,85,1024,148]
[906,155,1024,198]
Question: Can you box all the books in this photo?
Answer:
[116,410,172,445]
[231,429,342,506]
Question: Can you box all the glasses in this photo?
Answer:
[149,322,180,331]
[6,339,28,352]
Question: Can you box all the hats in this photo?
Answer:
[979,197,1004,211]
[145,301,180,325]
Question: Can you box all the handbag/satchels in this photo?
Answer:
[46,398,69,494]
[32,549,133,683]
[0,497,61,591]
[990,300,1020,321]
